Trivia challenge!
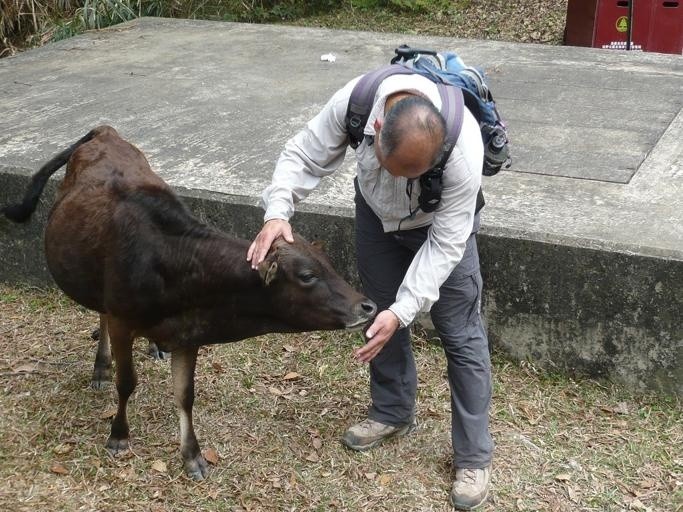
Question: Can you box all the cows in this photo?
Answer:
[1,124,382,485]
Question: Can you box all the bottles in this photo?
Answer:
[485,135,506,163]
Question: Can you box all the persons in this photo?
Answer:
[246,66,495,511]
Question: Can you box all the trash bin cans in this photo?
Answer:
[563,0,683,55]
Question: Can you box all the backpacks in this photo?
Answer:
[346,43,509,213]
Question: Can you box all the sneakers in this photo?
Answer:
[449,458,493,512]
[339,418,417,452]
